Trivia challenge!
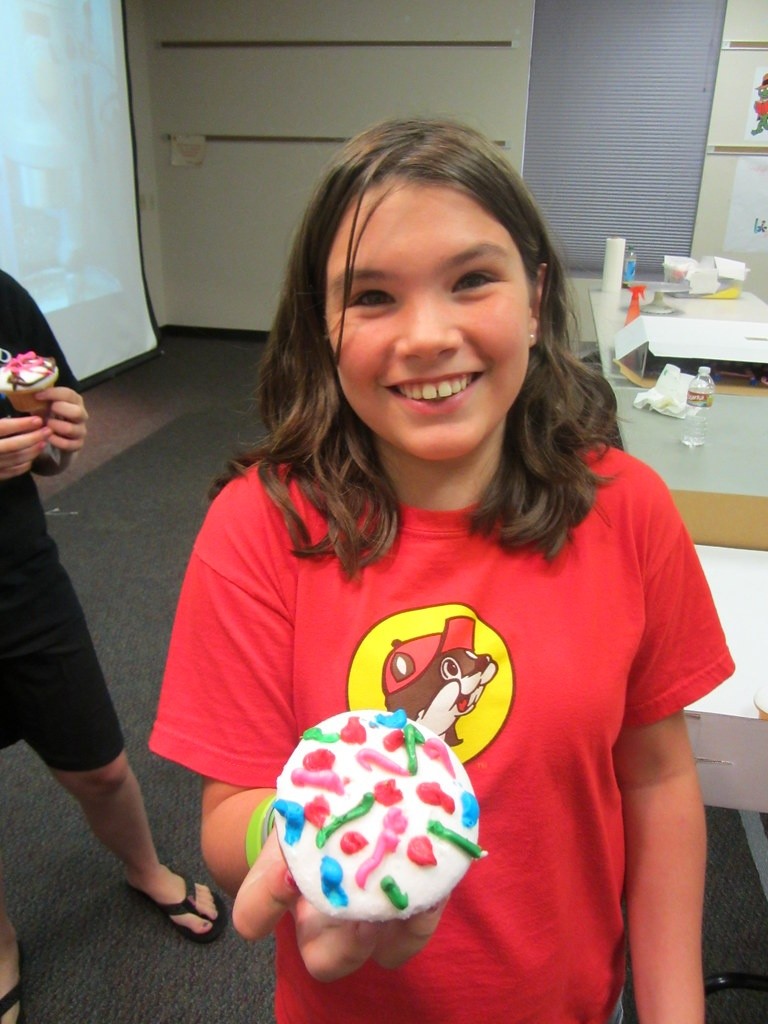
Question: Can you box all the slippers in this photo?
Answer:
[125,866,229,943]
[0,938,24,1024]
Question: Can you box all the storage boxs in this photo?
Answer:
[611,314,768,397]
[681,543,768,813]
[662,262,751,300]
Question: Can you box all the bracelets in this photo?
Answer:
[246,795,277,872]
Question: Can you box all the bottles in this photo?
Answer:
[622,246,636,287]
[680,365,715,446]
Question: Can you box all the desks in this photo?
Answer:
[587,286,768,495]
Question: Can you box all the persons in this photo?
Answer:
[1,267,227,1024]
[150,120,736,1024]
